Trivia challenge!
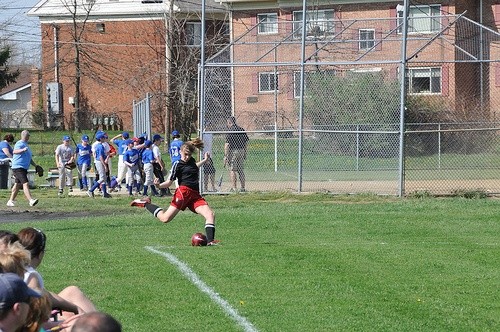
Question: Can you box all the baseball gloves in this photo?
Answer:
[35,166,43,177]
[109,144,116,157]
[64,162,76,170]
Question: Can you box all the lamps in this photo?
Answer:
[97,23,106,33]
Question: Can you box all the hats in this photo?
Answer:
[21,130,32,136]
[153,134,161,139]
[64,136,70,140]
[126,140,136,145]
[123,132,129,139]
[81,135,89,142]
[172,130,179,135]
[145,140,151,146]
[95,131,108,140]
[0,272,41,309]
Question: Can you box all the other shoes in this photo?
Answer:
[229,188,238,192]
[130,197,151,207]
[207,240,221,246]
[80,186,88,191]
[110,188,118,193]
[58,189,63,195]
[69,186,73,192]
[7,200,17,207]
[117,184,122,188]
[87,190,94,198]
[126,184,173,197]
[104,193,112,198]
[239,189,246,192]
[29,199,38,206]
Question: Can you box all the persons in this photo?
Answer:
[130,136,219,246]
[6,130,44,207]
[71,311,122,332]
[201,149,218,192]
[90,111,94,130]
[92,114,98,130]
[103,114,109,130]
[54,131,173,199]
[223,116,249,192]
[113,114,121,131]
[0,227,98,332]
[97,115,103,130]
[169,130,185,190]
[109,114,115,131]
[0,134,14,189]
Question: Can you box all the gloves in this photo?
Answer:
[36,165,44,177]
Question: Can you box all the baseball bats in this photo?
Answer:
[218,162,226,186]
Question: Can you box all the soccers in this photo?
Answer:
[192,232,207,246]
